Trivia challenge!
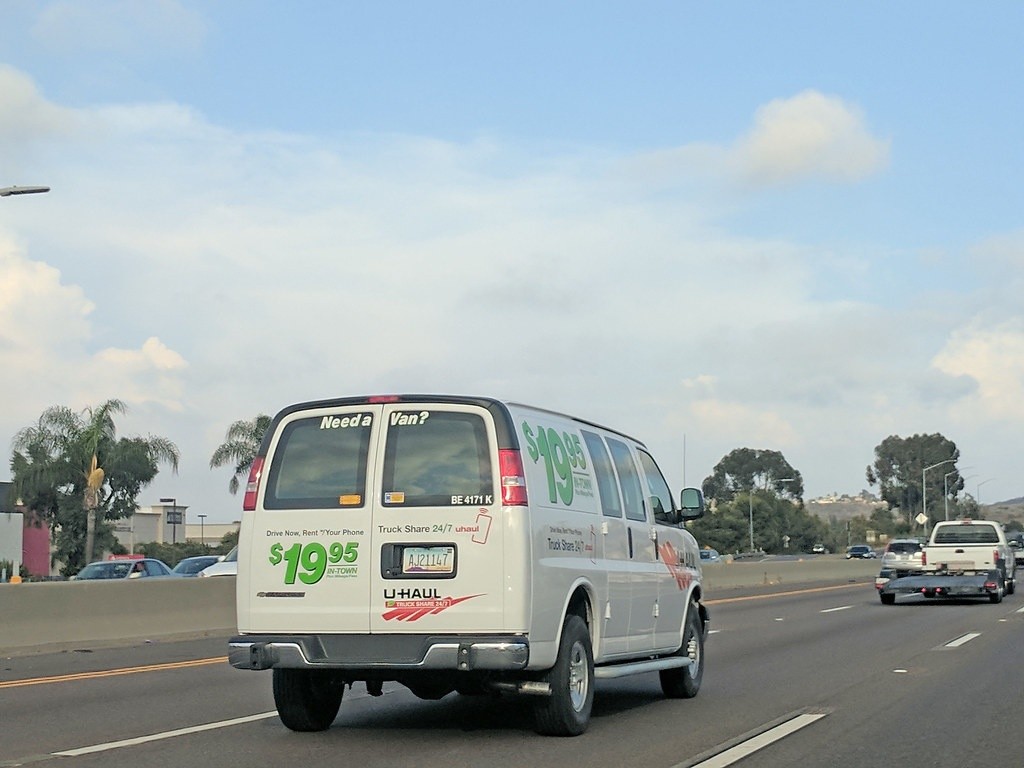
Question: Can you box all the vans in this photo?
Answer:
[226,392,711,737]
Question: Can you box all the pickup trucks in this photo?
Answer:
[875,520,1019,604]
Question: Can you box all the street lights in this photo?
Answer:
[749,478,796,552]
[922,459,959,535]
[198,514,208,543]
[943,464,976,521]
[977,477,996,518]
[159,498,176,544]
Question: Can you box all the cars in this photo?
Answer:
[699,548,720,562]
[174,555,225,577]
[845,545,877,559]
[811,544,824,554]
[72,560,180,580]
[879,537,926,572]
[197,543,238,577]
